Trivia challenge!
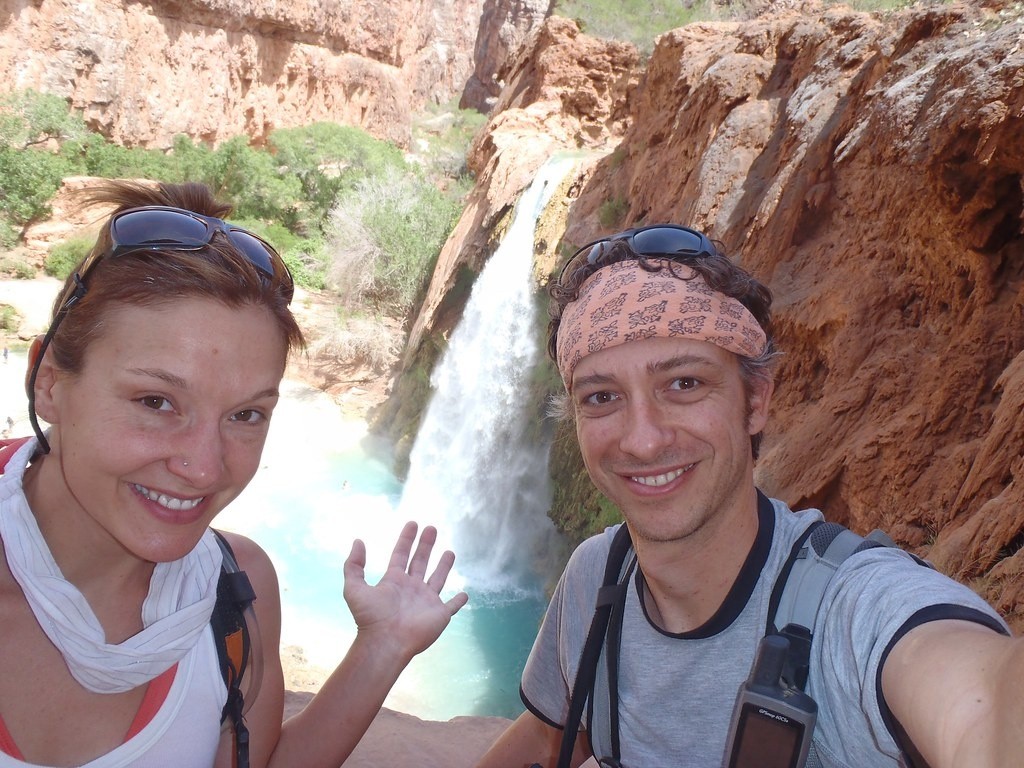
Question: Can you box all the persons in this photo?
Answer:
[473,222,1024,768]
[0,175,469,768]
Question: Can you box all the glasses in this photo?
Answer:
[54,204,293,317]
[556,225,719,317]
